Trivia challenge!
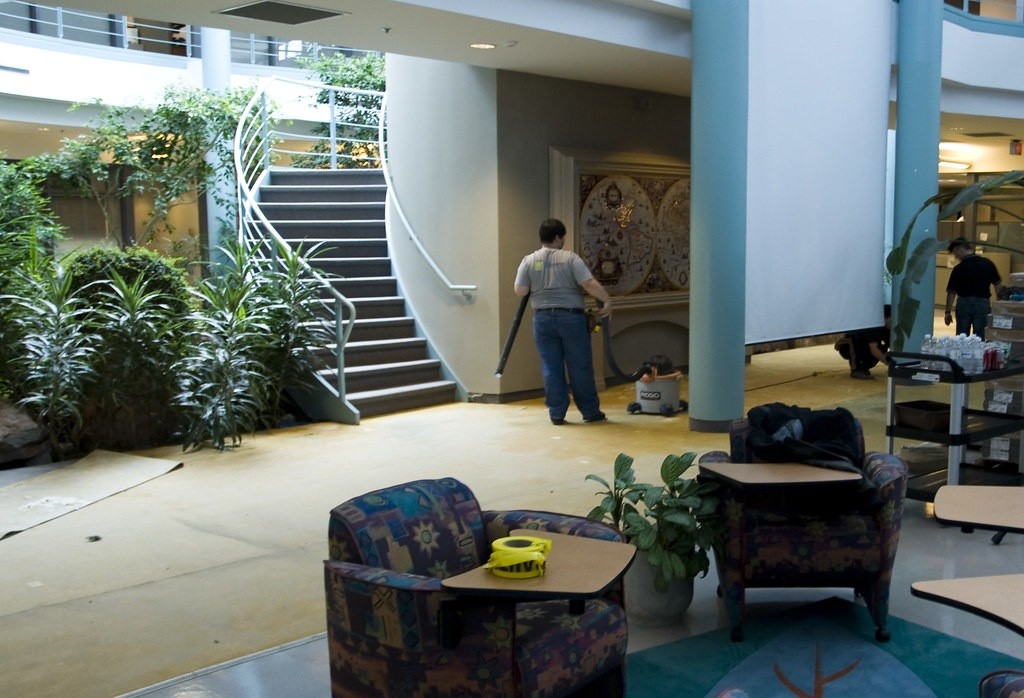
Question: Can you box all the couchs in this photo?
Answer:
[322,477,639,698]
[696,401,909,642]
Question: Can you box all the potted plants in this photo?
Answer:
[584,452,715,628]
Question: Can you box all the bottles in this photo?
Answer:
[921,332,984,375]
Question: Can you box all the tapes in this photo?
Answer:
[483,536,552,579]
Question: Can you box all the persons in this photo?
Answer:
[835,304,895,380]
[944,237,1003,342]
[514,218,612,424]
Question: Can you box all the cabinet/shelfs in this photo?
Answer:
[885,350,1024,527]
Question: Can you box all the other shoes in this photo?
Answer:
[583,412,605,420]
[552,418,563,425]
[850,370,873,380]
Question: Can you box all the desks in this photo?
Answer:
[438,527,637,598]
[934,485,1024,535]
[911,572,1024,636]
[699,460,864,488]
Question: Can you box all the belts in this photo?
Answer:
[550,307,584,313]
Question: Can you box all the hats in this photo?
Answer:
[947,237,965,252]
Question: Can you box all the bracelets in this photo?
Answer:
[946,311,950,313]
